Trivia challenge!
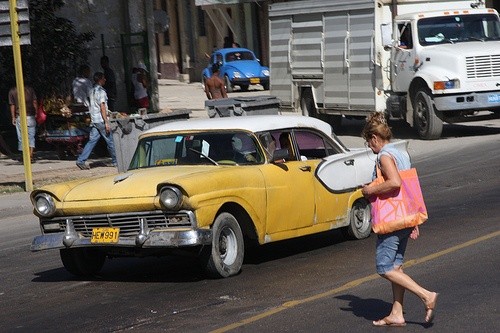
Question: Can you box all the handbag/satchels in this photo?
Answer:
[36,98,47,125]
[369,150,428,235]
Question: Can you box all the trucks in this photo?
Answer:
[266,0,500,141]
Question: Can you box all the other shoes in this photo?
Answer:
[30,157,36,162]
[76,161,89,170]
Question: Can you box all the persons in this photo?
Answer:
[9,41,293,164]
[361,110,440,326]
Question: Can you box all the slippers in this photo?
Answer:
[425,292,440,328]
[372,316,406,327]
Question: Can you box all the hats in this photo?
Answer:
[132,64,149,73]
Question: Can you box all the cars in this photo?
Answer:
[202,48,270,93]
[29,114,378,278]
